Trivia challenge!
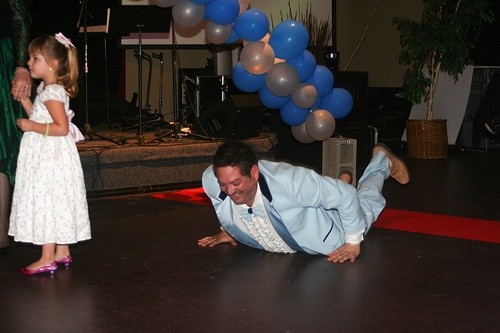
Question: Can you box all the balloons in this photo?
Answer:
[238,41,277,76]
[305,109,335,141]
[233,7,270,43]
[267,18,310,62]
[147,0,356,145]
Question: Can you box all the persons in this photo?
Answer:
[0,0,35,257]
[196,139,411,265]
[7,32,93,277]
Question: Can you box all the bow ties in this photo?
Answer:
[239,205,265,223]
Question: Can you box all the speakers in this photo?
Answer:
[191,94,265,140]
[330,71,369,132]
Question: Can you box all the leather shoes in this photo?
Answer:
[17,254,73,275]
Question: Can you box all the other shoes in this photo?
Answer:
[337,170,353,184]
[372,143,411,185]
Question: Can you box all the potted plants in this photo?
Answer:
[393,0,483,161]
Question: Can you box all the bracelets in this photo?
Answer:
[44,122,50,137]
[14,68,31,74]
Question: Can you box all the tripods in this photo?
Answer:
[76,0,215,147]
